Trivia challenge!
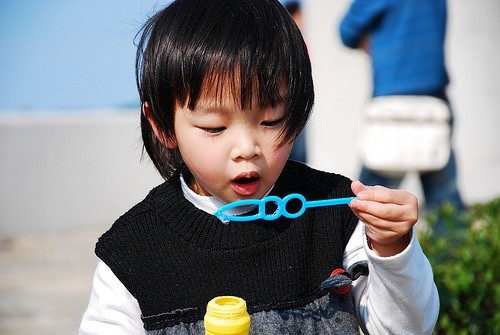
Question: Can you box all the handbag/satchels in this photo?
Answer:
[355,96,451,174]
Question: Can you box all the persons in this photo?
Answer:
[78,0,439,335]
[284,0,467,249]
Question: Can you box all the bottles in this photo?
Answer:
[204,296,250,335]
